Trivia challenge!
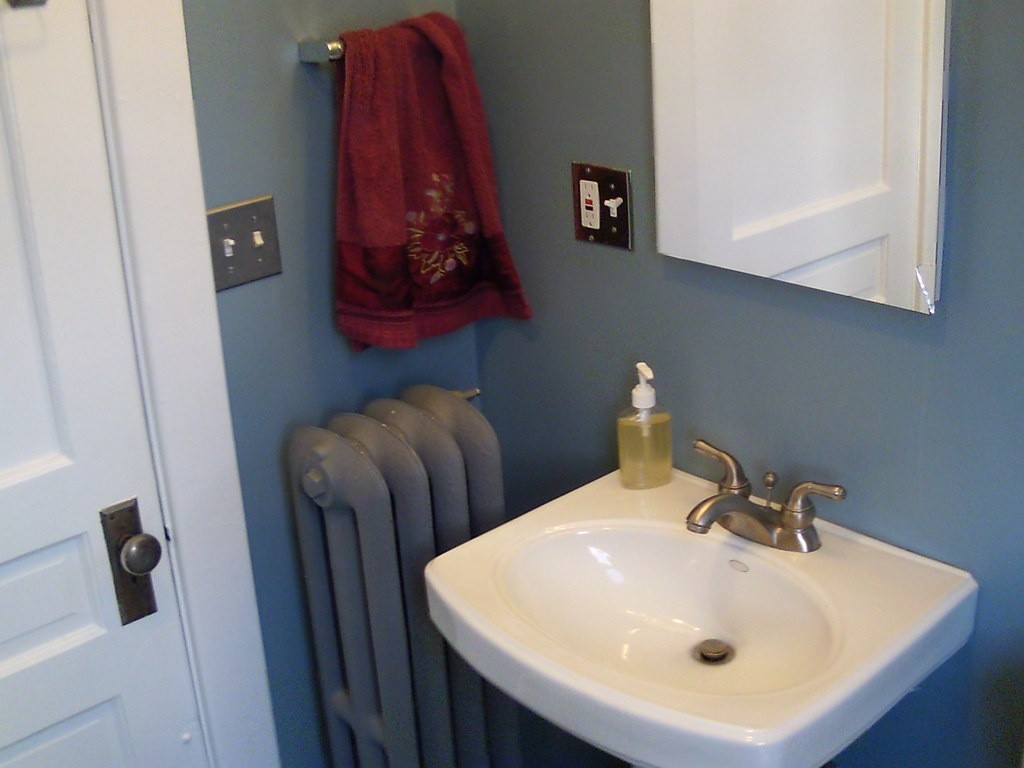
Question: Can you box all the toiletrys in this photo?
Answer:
[614,360,675,491]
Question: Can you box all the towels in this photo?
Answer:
[325,10,535,360]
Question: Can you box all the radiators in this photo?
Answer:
[290,381,529,767]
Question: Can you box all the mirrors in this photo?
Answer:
[646,0,946,315]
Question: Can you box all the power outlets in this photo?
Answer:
[571,162,634,250]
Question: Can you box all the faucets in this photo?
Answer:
[685,490,786,553]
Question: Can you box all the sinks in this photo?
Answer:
[423,455,979,768]
